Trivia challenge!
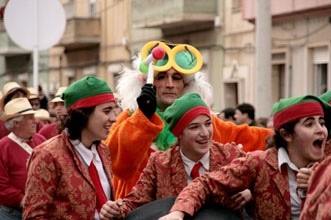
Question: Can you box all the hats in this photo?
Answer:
[163,93,211,137]
[318,89,331,104]
[61,75,116,112]
[26,87,40,99]
[0,98,39,122]
[0,82,30,112]
[50,86,68,102]
[272,90,331,131]
[33,109,53,124]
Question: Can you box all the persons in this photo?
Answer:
[0,81,269,142]
[0,97,46,219]
[158,94,331,219]
[99,92,251,220]
[104,40,275,202]
[21,75,117,220]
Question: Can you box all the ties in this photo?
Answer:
[87,162,108,213]
[190,162,203,180]
[292,170,307,211]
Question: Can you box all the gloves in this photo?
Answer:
[136,84,158,120]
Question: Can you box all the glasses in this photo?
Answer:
[142,40,204,75]
